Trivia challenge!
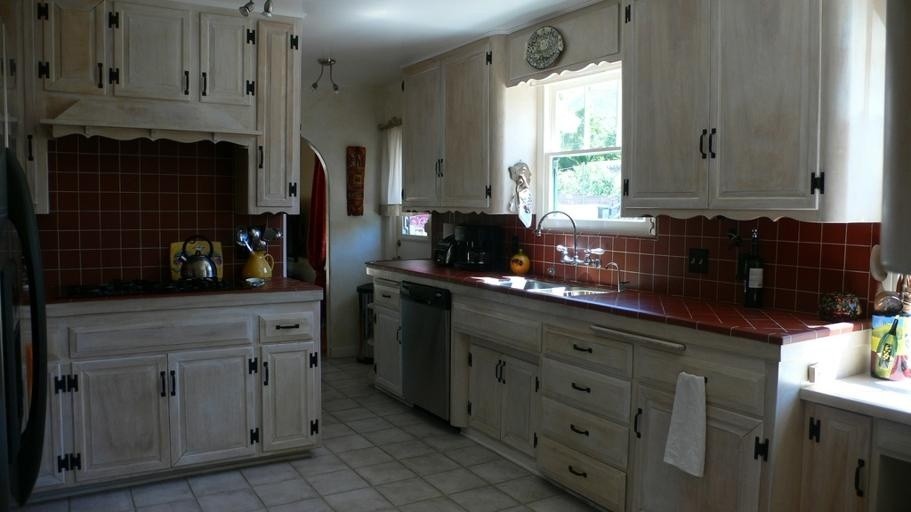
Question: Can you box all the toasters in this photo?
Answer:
[444,234,468,266]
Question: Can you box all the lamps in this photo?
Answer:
[310,57,341,94]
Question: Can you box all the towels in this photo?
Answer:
[662,369,709,479]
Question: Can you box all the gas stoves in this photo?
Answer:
[81,278,228,295]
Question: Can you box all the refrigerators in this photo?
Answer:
[0,150,50,510]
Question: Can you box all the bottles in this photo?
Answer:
[874,318,900,379]
[597,197,621,220]
[510,248,532,276]
[743,228,766,307]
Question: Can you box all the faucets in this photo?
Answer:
[604,261,621,293]
[534,211,580,261]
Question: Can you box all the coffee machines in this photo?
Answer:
[466,225,505,273]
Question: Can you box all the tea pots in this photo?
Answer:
[240,249,275,283]
[180,234,218,279]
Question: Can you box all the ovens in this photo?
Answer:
[394,280,452,420]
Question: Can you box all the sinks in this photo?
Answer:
[502,280,553,291]
[562,289,601,297]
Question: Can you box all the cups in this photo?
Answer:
[455,226,466,241]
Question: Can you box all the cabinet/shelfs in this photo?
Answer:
[452,285,537,471]
[537,295,633,502]
[46,1,114,137]
[15,306,65,503]
[65,295,258,488]
[619,2,893,225]
[802,402,883,511]
[258,291,321,460]
[401,42,535,215]
[628,311,874,511]
[371,280,402,395]
[246,11,300,216]
[113,1,261,144]
[0,1,49,212]
